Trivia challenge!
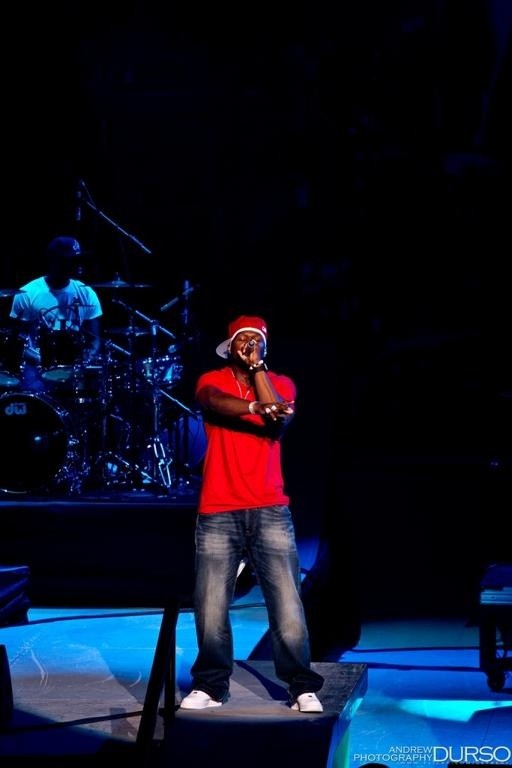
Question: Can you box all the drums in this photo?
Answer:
[36,328,94,383]
[1,332,29,387]
[1,387,76,496]
[118,334,183,388]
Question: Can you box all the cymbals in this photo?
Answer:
[1,289,26,297]
[82,281,154,288]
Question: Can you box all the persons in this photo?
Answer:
[1,238,105,495]
[180,315,324,713]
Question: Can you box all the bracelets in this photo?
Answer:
[249,360,263,370]
[249,401,258,414]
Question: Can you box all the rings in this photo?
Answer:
[265,407,271,413]
[270,405,277,411]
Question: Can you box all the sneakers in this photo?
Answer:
[180,689,222,709]
[291,692,323,712]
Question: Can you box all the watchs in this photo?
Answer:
[249,363,268,374]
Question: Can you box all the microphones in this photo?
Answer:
[76,180,86,221]
[244,341,255,356]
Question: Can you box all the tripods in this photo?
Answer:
[48,290,202,499]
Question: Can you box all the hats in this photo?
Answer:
[216,316,268,359]
